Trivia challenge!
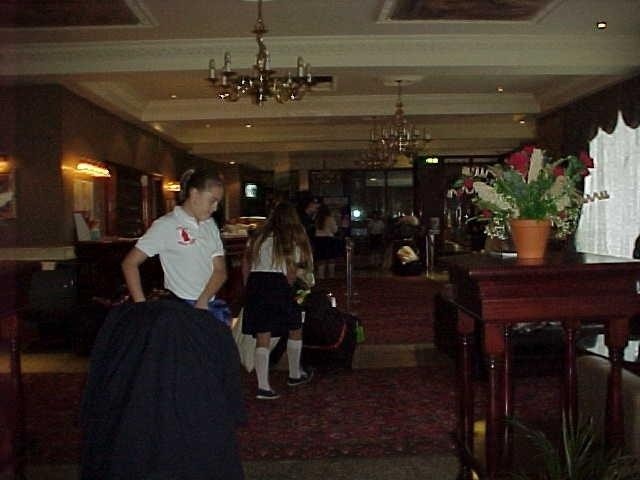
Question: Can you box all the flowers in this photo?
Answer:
[466,151,610,239]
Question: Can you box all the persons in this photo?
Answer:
[314,202,338,280]
[366,209,387,264]
[121,166,234,327]
[239,200,316,401]
[294,189,320,235]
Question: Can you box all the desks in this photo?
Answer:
[443,254,639,480]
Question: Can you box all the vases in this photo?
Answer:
[510,220,553,265]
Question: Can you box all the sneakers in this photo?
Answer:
[255,388,281,400]
[285,368,314,387]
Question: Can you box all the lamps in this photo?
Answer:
[203,0,311,106]
[373,79,431,158]
